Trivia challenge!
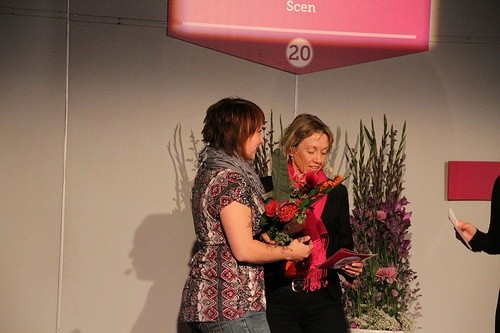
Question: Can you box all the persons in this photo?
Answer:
[453,175,500,333]
[261,114,364,333]
[179,97,313,333]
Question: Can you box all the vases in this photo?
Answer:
[346,328,416,333]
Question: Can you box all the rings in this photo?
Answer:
[352,271,355,274]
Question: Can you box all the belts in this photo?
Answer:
[270,279,306,292]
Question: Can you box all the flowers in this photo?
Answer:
[335,112,424,333]
